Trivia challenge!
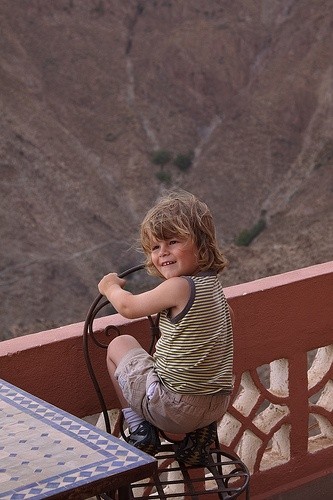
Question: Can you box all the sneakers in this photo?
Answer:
[125,420,161,456]
[173,419,217,468]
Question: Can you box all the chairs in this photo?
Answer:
[82,262,251,500]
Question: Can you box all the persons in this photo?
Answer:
[98,195,235,457]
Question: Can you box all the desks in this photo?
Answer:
[0,377,159,500]
